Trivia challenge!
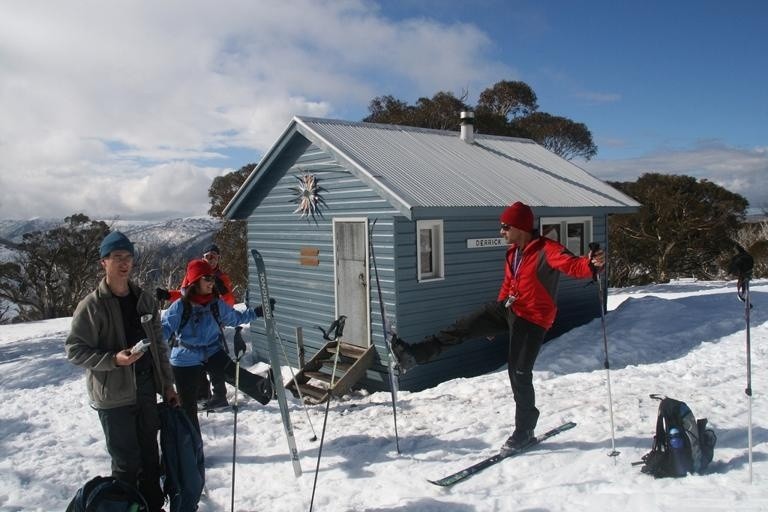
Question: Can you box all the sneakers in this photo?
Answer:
[504,427,532,448]
[262,368,278,399]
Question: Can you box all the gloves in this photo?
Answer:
[155,287,171,300]
[255,298,276,319]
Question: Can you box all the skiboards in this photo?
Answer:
[426,421,577,486]
[251,249,302,479]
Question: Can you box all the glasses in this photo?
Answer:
[202,274,214,282]
[104,255,134,262]
[500,223,512,230]
[204,251,218,260]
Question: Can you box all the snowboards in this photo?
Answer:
[221,354,273,406]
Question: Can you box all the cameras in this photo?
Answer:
[130,338,151,354]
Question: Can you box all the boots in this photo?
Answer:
[207,388,230,408]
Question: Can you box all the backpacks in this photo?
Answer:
[631,399,717,478]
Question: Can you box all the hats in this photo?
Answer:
[186,260,213,284]
[100,232,134,259]
[500,201,534,233]
[202,244,219,255]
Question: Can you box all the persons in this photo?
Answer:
[156,244,235,408]
[66,230,180,511]
[162,256,276,432]
[495,201,605,449]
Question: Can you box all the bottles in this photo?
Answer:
[669,428,690,474]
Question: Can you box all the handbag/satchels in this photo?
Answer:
[159,398,206,512]
[66,474,150,511]
